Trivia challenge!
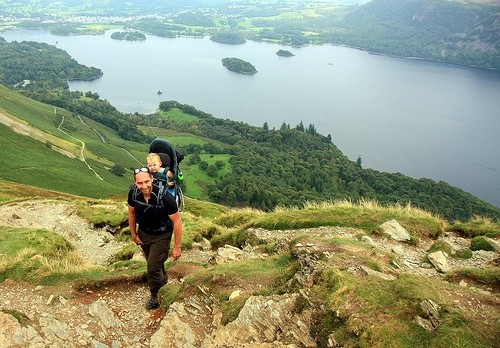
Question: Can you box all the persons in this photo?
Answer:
[128,167,183,310]
[146,152,179,208]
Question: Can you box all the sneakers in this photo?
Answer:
[146,298,158,309]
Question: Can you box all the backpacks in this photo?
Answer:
[148,138,184,180]
[152,173,176,198]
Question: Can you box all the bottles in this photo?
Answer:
[178,172,184,184]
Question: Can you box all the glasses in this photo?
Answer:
[134,167,148,174]
